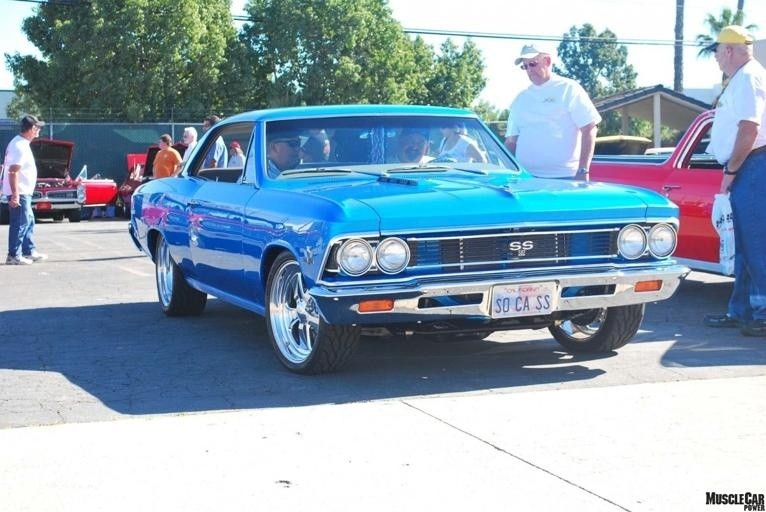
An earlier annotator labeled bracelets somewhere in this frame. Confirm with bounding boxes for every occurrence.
[722,162,739,175]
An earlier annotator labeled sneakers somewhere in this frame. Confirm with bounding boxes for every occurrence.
[741,319,766,337]
[21,251,47,262]
[703,311,752,328]
[6,255,33,265]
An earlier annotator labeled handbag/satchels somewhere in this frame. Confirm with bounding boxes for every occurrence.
[80,205,124,220]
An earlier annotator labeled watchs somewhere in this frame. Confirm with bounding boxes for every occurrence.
[577,167,589,175]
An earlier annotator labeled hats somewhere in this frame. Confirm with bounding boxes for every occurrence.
[515,43,551,65]
[229,142,240,149]
[705,25,753,50]
[20,115,45,130]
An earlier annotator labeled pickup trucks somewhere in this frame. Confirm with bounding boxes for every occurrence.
[575,107,735,279]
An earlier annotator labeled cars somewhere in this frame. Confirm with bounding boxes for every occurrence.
[129,104,690,376]
[116,142,189,219]
[0,137,117,222]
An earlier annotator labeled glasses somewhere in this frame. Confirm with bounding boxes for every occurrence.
[520,57,538,70]
[273,139,301,149]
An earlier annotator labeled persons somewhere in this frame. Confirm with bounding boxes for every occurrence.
[504,39,600,182]
[2,115,50,266]
[229,141,246,169]
[201,115,226,168]
[387,123,429,166]
[152,134,184,179]
[703,24,766,336]
[239,120,370,185]
[437,118,489,165]
[182,127,204,172]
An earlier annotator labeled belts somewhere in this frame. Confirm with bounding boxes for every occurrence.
[751,145,766,155]
